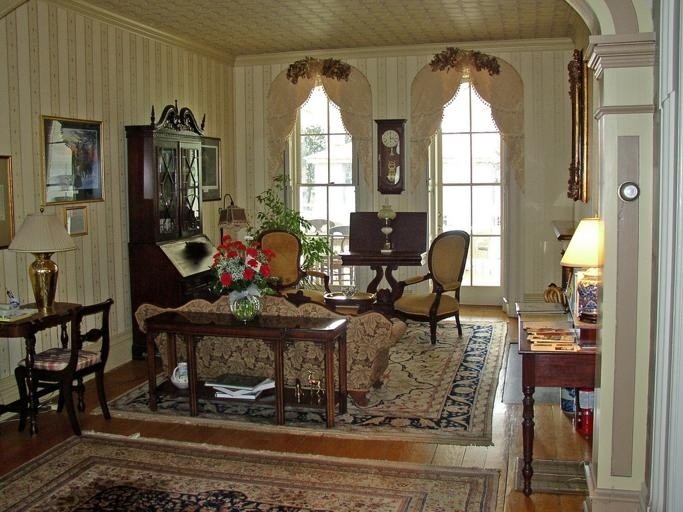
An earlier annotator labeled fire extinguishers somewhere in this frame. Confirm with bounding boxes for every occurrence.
[574,387,594,441]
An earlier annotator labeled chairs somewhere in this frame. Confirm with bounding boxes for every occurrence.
[393,231,470,344]
[15,299,114,436]
[257,229,331,304]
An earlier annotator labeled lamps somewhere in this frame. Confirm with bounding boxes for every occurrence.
[7,209,79,308]
[219,194,247,246]
[560,213,604,323]
[378,200,396,255]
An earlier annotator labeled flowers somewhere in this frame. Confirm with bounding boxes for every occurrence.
[208,235,278,322]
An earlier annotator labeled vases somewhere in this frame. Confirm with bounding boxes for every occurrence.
[229,285,262,323]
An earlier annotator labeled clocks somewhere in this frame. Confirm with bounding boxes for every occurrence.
[375,119,408,195]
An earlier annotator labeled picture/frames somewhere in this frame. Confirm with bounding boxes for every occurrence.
[0,154,15,249]
[201,137,222,201]
[566,50,588,203]
[41,114,106,206]
[64,205,89,237]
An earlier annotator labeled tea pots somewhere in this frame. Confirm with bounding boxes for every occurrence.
[171,362,188,389]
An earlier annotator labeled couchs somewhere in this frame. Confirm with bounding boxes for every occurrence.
[136,290,407,407]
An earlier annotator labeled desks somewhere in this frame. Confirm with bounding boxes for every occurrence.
[517,314,601,496]
[1,302,81,439]
[145,311,347,427]
[338,211,428,294]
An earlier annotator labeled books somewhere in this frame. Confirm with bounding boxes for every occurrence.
[205,372,277,401]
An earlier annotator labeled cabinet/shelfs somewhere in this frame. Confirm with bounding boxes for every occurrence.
[159,140,202,241]
[183,270,219,303]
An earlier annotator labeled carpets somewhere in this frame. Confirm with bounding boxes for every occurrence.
[88,321,507,445]
[0,431,501,512]
